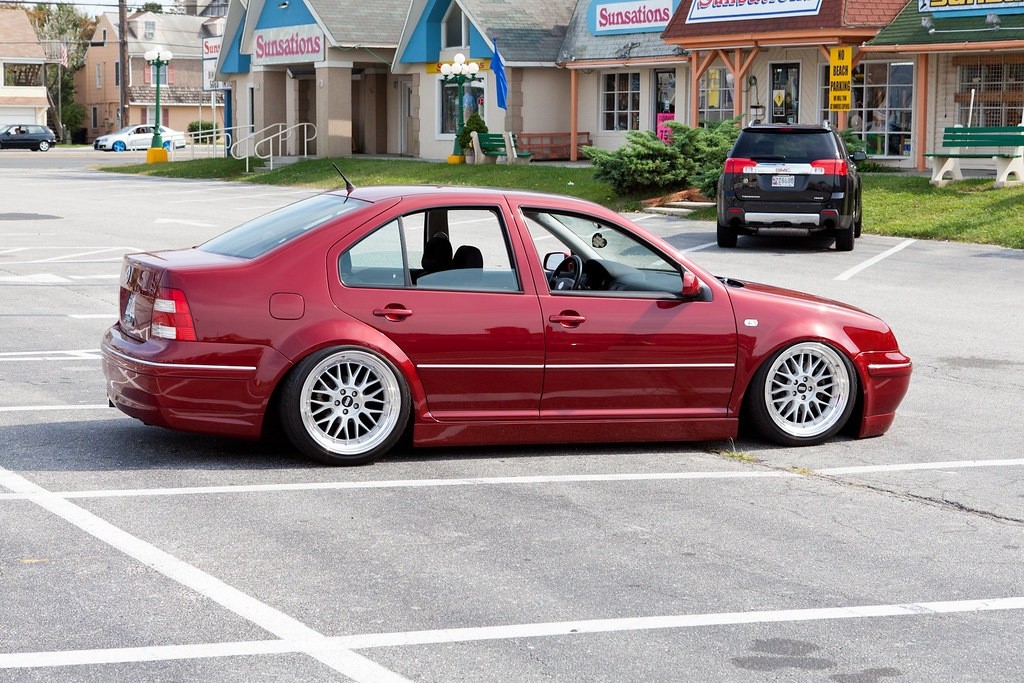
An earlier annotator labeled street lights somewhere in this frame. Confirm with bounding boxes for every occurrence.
[440,54,479,168]
[142,50,174,146]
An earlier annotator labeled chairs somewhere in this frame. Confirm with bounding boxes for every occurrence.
[417,232,453,280]
[20,131,25,134]
[453,245,483,285]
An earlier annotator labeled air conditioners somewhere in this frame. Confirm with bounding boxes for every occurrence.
[145,31,154,38]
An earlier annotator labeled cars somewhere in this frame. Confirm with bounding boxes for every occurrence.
[93,122,188,152]
[101,184,914,466]
[0,123,58,152]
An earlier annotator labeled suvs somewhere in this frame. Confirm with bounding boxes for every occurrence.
[716,118,866,252]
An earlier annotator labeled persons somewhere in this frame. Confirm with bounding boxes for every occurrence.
[140,129,145,134]
[15,128,21,134]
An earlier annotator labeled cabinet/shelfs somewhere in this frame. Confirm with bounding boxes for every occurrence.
[518,132,592,159]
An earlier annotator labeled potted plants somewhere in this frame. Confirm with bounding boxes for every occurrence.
[459,113,489,164]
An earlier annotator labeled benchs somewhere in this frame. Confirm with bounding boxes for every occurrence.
[470,131,535,165]
[285,226,365,284]
[922,124,1024,188]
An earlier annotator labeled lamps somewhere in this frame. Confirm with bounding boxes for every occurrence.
[562,51,576,62]
[920,17,936,35]
[277,1,288,8]
[985,14,1002,31]
